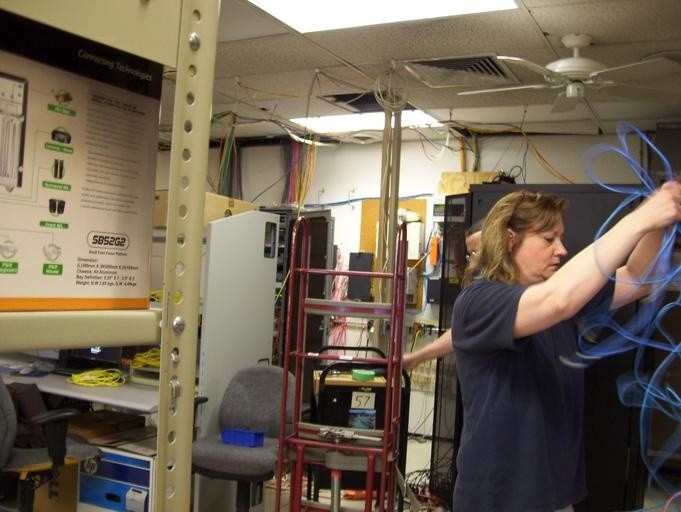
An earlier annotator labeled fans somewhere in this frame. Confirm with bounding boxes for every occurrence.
[458,32,681,114]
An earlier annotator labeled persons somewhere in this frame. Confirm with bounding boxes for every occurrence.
[451,180,680,512]
[401,216,486,371]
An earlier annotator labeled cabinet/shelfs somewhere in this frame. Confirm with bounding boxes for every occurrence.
[77,424,159,511]
[306,346,411,511]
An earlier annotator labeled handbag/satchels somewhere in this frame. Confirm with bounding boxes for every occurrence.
[7,382,49,449]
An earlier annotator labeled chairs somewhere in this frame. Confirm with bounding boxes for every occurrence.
[0,376,101,512]
[193,365,296,512]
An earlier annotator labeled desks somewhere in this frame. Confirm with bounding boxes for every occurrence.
[0,366,158,415]
